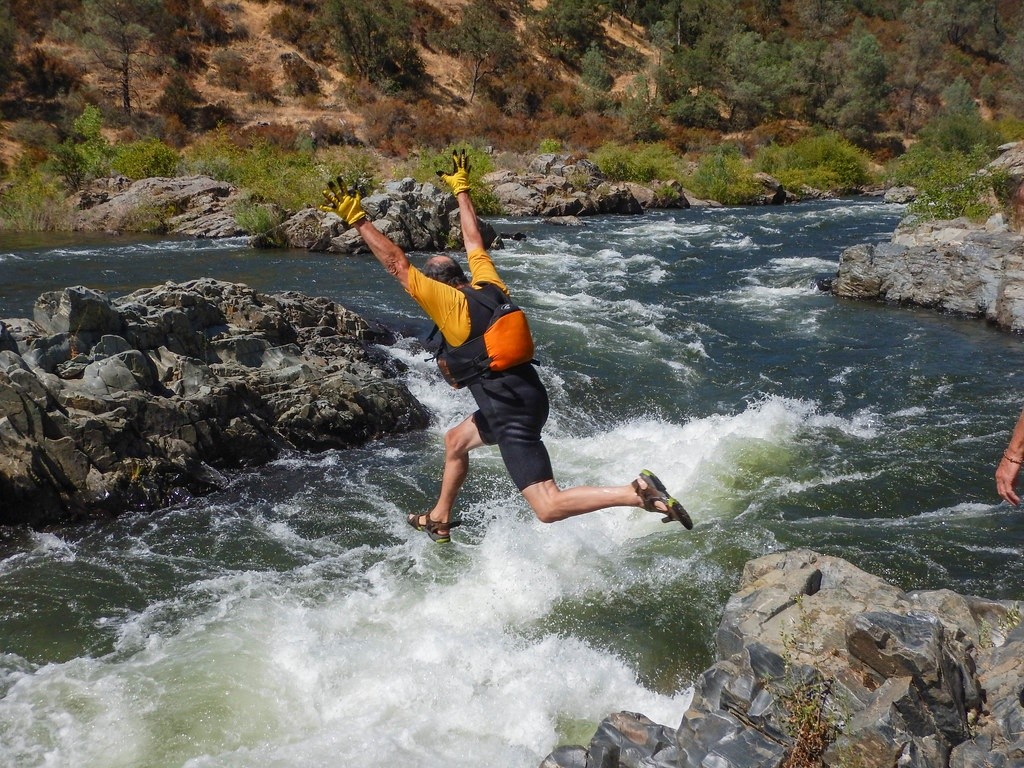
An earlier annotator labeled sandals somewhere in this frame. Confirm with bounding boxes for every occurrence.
[407,513,451,544]
[631,469,692,530]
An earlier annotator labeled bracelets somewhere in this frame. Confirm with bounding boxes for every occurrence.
[1004,448,1024,464]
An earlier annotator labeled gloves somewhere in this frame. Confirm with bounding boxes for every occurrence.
[436,148,472,196]
[319,177,367,226]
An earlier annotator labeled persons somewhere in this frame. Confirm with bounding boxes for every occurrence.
[320,151,693,545]
[994,407,1024,507]
